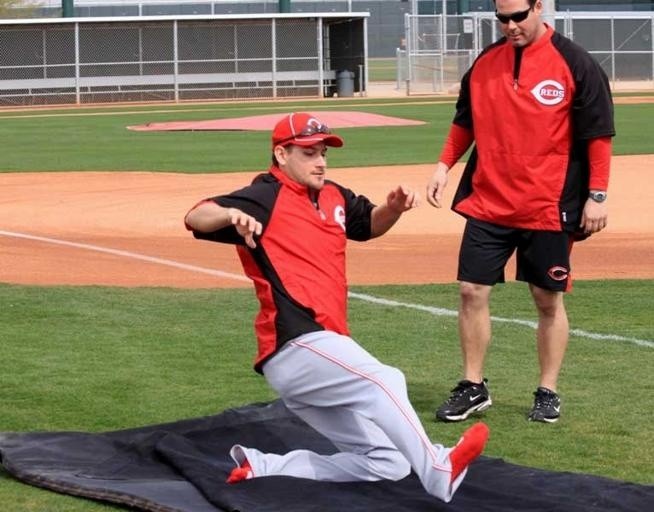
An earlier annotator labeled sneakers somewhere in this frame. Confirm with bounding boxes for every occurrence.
[436,379,492,420]
[529,386,560,422]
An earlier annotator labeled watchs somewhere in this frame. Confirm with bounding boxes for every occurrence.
[589,192,607,202]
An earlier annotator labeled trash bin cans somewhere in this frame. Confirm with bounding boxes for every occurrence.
[336,69,355,97]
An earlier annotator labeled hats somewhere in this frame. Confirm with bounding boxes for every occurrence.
[272,112,343,151]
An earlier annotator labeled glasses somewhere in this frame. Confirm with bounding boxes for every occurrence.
[496,7,532,24]
[274,124,330,144]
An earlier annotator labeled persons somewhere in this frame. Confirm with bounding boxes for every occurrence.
[184,112,491,502]
[426,0,616,422]
